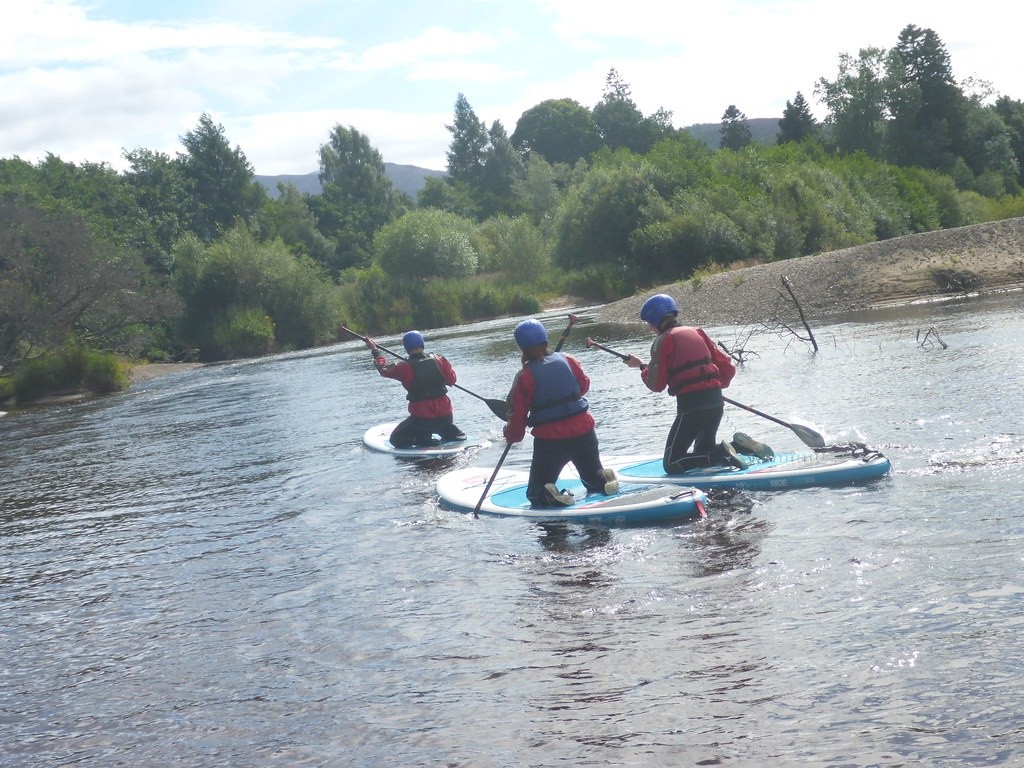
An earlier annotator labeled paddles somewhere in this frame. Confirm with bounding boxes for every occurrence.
[472,313,580,518]
[584,336,826,451]
[338,323,509,422]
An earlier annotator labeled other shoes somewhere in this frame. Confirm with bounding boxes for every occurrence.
[416,431,440,447]
[442,425,466,440]
[597,469,619,495]
[544,483,576,506]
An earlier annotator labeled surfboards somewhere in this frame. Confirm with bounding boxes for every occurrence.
[435,466,708,524]
[362,421,467,457]
[556,448,893,491]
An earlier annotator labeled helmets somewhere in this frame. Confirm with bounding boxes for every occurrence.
[514,319,548,350]
[402,330,425,354]
[640,294,679,327]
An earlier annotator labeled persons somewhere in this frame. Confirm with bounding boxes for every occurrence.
[503,318,618,506]
[362,330,467,449]
[622,293,773,470]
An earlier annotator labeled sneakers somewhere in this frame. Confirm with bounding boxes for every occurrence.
[731,432,775,461]
[715,439,749,471]
